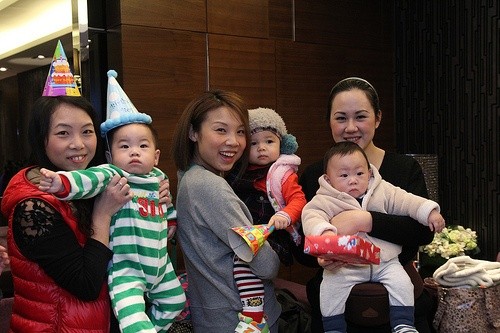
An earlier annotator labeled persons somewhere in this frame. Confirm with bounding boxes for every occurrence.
[298,74,445,333]
[169,88,307,333]
[2,39,187,333]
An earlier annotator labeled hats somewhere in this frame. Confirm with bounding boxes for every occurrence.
[101,68,153,136]
[246,106,298,154]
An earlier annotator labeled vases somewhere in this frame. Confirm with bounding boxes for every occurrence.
[426,254,456,278]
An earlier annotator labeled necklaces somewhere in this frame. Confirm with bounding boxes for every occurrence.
[167,191,170,196]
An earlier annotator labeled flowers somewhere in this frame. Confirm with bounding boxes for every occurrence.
[424,225,480,261]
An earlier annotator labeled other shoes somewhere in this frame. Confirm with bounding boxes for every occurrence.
[234,313,270,333]
[394,323,418,333]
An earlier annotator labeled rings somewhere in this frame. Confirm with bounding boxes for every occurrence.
[119,181,123,186]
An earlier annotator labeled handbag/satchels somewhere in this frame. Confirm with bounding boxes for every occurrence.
[422,277,500,332]
